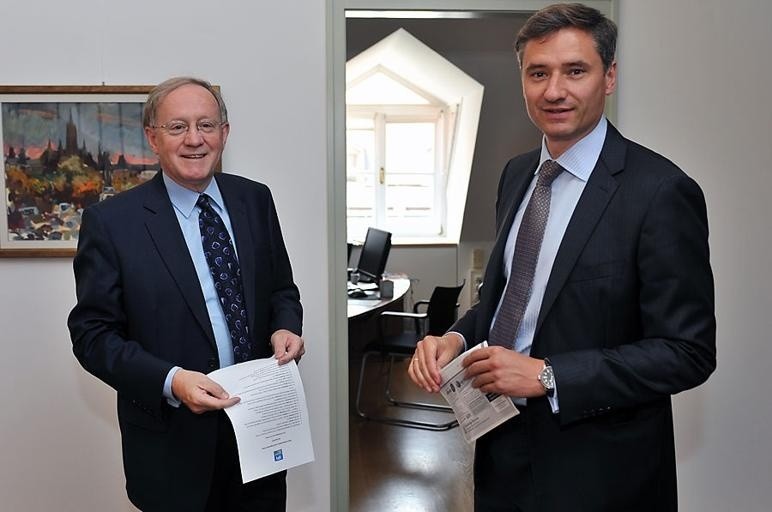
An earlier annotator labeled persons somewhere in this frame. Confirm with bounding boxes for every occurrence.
[67,78,306,512]
[407,3,716,512]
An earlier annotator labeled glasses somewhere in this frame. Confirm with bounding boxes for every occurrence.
[153,118,226,135]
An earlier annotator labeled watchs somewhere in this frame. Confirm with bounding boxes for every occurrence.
[537,358,554,397]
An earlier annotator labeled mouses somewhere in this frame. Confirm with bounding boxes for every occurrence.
[349,288,366,298]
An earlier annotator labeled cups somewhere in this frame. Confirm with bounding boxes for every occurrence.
[381,279,394,298]
[351,273,359,285]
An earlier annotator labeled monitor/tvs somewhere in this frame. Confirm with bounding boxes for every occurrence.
[357,227,392,292]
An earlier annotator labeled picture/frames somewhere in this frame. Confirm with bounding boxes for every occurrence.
[0,85,221,258]
[468,268,484,308]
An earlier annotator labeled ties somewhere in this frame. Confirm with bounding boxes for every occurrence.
[196,194,253,364]
[488,160,565,351]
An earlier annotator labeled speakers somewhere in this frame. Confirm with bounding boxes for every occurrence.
[379,280,393,298]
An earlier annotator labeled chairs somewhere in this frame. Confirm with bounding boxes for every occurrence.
[355,278,470,431]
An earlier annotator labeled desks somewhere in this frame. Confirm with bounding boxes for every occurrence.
[347,273,410,360]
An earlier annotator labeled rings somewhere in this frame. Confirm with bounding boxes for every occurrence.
[413,358,419,361]
